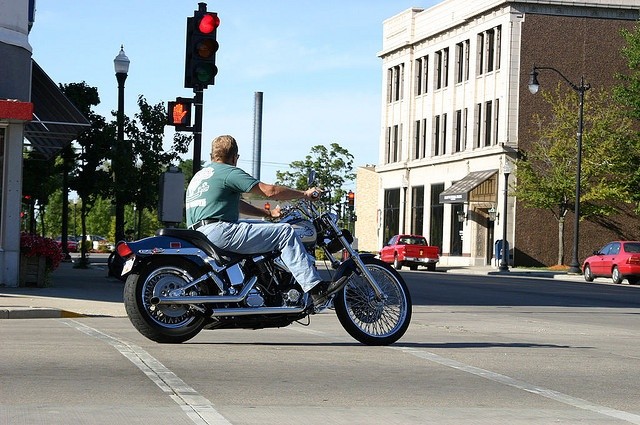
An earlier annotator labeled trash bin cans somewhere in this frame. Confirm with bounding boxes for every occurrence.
[495,239,509,267]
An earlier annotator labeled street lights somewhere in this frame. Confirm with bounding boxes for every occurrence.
[499,160,510,272]
[528,61,591,274]
[108,43,130,275]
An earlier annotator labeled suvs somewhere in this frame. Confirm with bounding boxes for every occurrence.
[77,235,116,251]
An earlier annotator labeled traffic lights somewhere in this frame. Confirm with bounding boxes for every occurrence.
[185,9,220,87]
[264,203,270,211]
[168,100,191,125]
[348,191,354,209]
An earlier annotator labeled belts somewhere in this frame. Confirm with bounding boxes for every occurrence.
[189,219,230,230]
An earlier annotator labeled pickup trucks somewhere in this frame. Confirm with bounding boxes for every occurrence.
[379,234,440,270]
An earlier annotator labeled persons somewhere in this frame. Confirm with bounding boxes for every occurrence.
[186,135,350,308]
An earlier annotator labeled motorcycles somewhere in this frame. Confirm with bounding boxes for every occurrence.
[116,170,412,344]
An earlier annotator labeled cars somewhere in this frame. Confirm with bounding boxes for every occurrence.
[56,236,78,252]
[582,240,640,284]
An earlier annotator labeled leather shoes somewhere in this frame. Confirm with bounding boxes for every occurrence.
[309,276,348,306]
